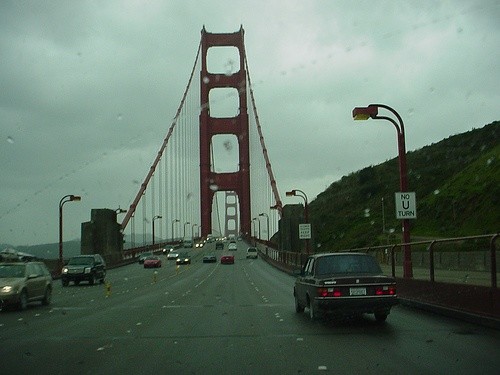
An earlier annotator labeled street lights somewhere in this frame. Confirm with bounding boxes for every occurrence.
[59,194,81,259]
[172,220,180,242]
[251,221,255,237]
[192,224,197,238]
[253,218,260,240]
[152,216,162,245]
[259,213,270,242]
[198,225,201,238]
[285,189,309,254]
[184,222,190,236]
[352,103,414,278]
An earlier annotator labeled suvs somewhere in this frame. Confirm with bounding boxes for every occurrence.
[61,254,106,287]
[247,247,258,258]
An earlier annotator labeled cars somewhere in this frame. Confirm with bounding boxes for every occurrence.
[144,256,162,267]
[139,252,153,263]
[0,260,54,311]
[184,234,243,264]
[163,244,191,264]
[294,252,398,325]
